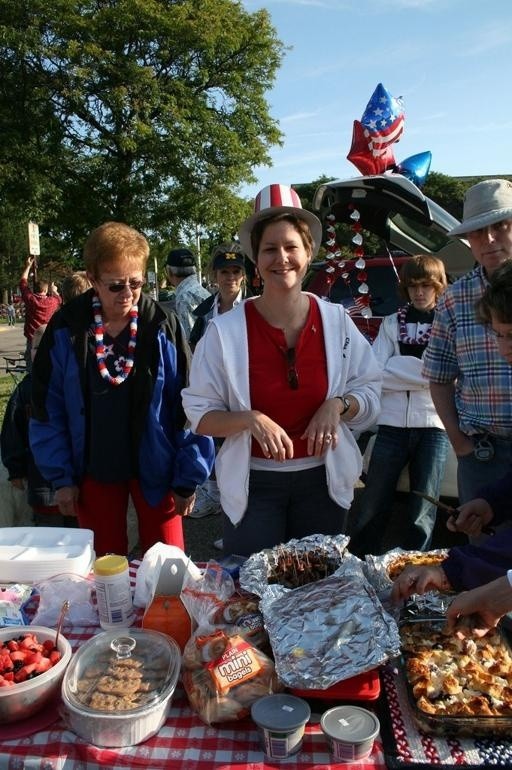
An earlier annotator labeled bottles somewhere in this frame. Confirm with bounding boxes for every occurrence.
[94,555,137,632]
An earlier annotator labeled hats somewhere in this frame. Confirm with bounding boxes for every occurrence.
[214,252,245,270]
[239,184,323,264]
[162,249,196,268]
[446,178,512,236]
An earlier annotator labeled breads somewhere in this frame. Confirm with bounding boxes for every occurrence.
[401,619,512,738]
[184,634,284,724]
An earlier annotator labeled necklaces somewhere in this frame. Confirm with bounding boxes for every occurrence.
[399,305,432,346]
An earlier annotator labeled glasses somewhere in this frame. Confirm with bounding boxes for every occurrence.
[287,347,298,388]
[98,276,147,292]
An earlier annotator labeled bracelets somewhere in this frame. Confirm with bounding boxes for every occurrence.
[334,396,351,414]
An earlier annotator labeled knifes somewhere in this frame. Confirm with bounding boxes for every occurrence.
[411,487,499,539]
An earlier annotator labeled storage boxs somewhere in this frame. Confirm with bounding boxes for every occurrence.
[285,670,381,714]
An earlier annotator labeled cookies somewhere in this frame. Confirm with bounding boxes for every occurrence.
[76,645,170,712]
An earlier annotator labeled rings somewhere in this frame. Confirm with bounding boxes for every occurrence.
[324,434,332,440]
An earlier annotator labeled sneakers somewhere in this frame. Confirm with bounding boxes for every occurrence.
[189,493,221,518]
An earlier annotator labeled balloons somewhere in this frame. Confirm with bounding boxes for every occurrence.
[361,83,406,158]
[391,150,432,191]
[346,119,397,177]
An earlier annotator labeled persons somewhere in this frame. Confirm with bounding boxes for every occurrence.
[29,221,216,557]
[6,259,93,370]
[347,252,448,557]
[422,178,511,550]
[392,258,512,602]
[440,569,512,643]
[2,325,56,509]
[156,249,246,519]
[180,186,384,564]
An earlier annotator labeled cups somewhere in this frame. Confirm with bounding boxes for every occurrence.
[320,705,378,761]
[250,693,311,759]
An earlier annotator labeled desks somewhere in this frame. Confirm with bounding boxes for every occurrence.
[0,559,512,769]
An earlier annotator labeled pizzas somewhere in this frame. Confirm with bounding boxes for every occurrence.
[388,554,447,577]
